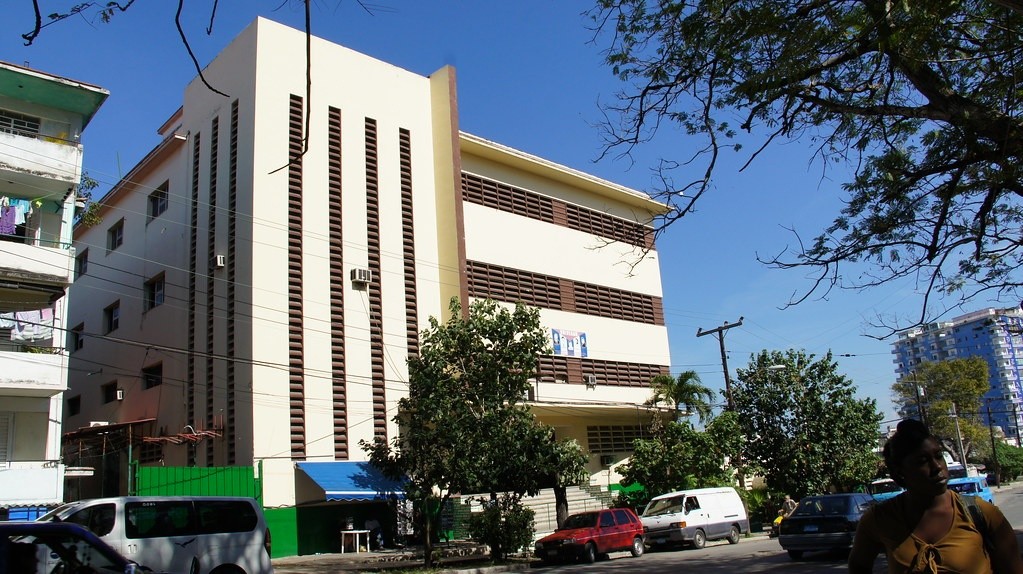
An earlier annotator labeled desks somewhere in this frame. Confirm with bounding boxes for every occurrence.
[341,529,371,554]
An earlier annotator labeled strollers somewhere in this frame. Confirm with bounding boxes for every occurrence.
[768,510,790,537]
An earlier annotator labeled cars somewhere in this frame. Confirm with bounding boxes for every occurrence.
[870,478,907,503]
[780,494,880,559]
[534,507,646,564]
[0,521,153,574]
[947,477,995,507]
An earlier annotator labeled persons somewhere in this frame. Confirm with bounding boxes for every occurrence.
[847,418,1023,574]
[783,495,796,515]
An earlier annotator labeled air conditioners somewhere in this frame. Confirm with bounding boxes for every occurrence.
[210,255,224,269]
[604,457,616,466]
[90,422,109,434]
[351,267,371,283]
[589,376,596,385]
[116,390,122,400]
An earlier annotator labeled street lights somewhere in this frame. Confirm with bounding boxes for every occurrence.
[949,414,969,477]
[916,387,927,421]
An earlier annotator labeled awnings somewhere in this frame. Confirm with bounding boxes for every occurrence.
[296,461,425,502]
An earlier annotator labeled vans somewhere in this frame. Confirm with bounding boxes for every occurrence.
[639,486,749,549]
[948,465,987,490]
[10,494,273,574]
